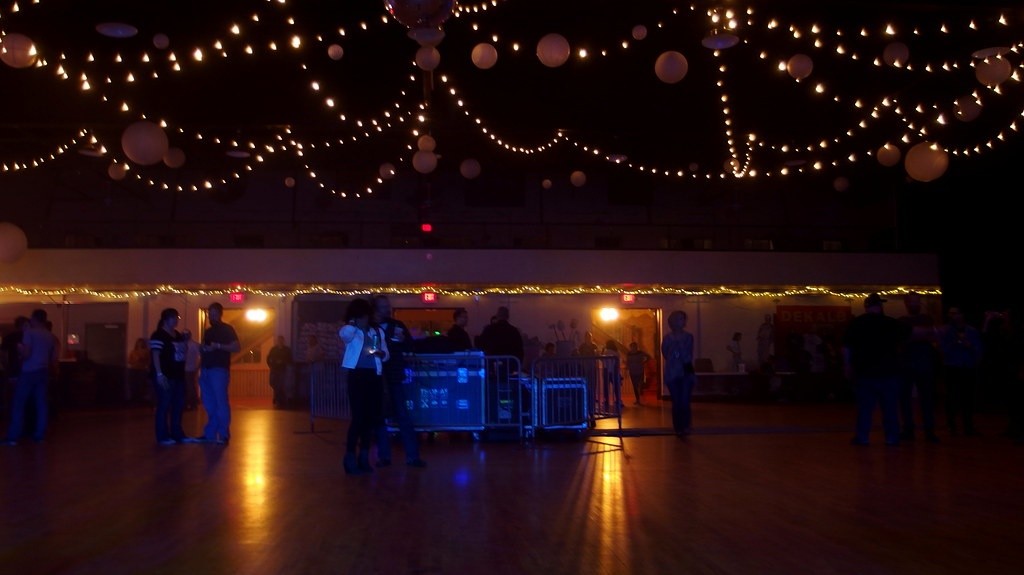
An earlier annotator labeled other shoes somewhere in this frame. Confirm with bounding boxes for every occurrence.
[850,439,867,445]
[216,439,225,445]
[406,459,427,468]
[193,437,217,444]
[949,427,958,438]
[157,438,177,447]
[0,438,17,447]
[900,424,916,441]
[375,459,391,467]
[923,425,939,444]
[963,421,984,438]
[177,436,192,443]
[343,449,375,474]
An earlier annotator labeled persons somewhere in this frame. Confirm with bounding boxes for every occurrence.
[445,306,524,382]
[266,335,293,408]
[303,335,326,364]
[661,311,696,440]
[541,332,652,409]
[726,331,743,370]
[842,293,986,445]
[128,302,241,446]
[338,295,428,475]
[0,309,60,447]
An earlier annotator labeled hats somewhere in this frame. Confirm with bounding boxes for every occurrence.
[864,293,882,307]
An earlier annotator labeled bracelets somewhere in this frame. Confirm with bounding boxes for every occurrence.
[157,373,162,376]
[217,344,220,349]
